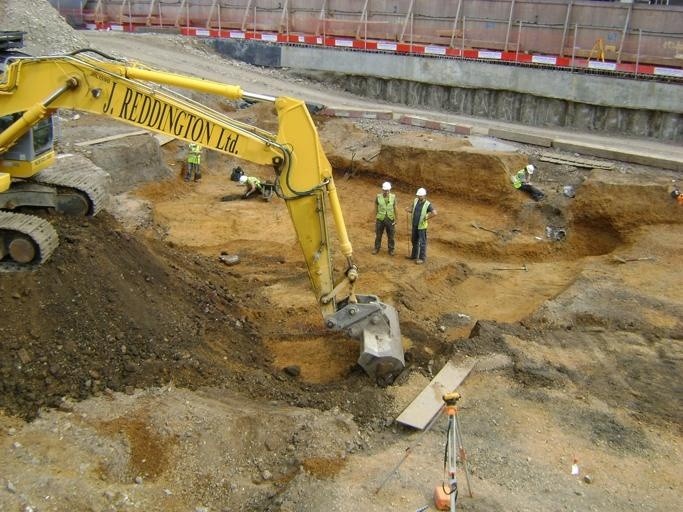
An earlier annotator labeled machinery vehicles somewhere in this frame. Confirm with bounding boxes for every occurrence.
[0,28,406,388]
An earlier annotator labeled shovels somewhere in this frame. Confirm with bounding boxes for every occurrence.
[362,151,380,163]
[194,155,201,181]
[614,255,652,263]
[471,221,498,233]
[343,152,356,181]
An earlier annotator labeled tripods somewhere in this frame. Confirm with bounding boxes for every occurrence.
[375,405,472,512]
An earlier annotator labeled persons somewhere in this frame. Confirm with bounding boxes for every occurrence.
[183,143,203,182]
[404,187,436,265]
[514,164,548,201]
[371,182,398,255]
[240,175,262,197]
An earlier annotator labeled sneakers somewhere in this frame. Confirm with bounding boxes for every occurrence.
[416,258,424,264]
[371,248,379,254]
[406,256,417,259]
[388,249,394,256]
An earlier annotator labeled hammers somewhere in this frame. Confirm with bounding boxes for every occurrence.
[493,264,528,272]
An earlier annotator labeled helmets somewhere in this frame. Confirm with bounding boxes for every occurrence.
[383,182,391,191]
[416,188,426,196]
[526,164,534,175]
[240,175,247,184]
